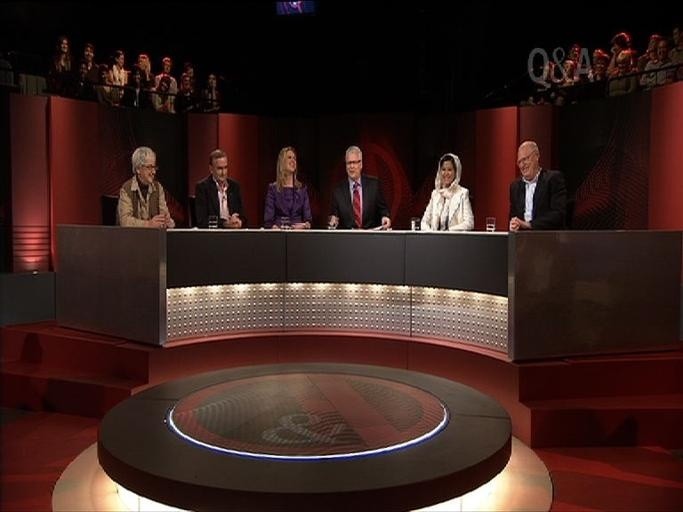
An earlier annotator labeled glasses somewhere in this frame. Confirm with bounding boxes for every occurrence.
[141,164,158,170]
[346,159,361,166]
[517,150,536,166]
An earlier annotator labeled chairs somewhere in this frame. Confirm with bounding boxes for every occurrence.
[100,194,119,225]
[189,195,196,227]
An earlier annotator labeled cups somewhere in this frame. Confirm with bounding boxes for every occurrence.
[485,216,495,230]
[208,216,218,228]
[326,214,337,229]
[280,216,292,229]
[411,216,420,230]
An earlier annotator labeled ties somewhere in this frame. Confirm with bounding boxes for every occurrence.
[220,187,228,219]
[353,181,362,229]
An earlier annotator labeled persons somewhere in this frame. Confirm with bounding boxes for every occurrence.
[524,26,681,106]
[114,147,177,230]
[194,150,247,228]
[51,36,219,111]
[325,144,392,230]
[262,147,314,230]
[507,141,568,231]
[416,153,476,234]
